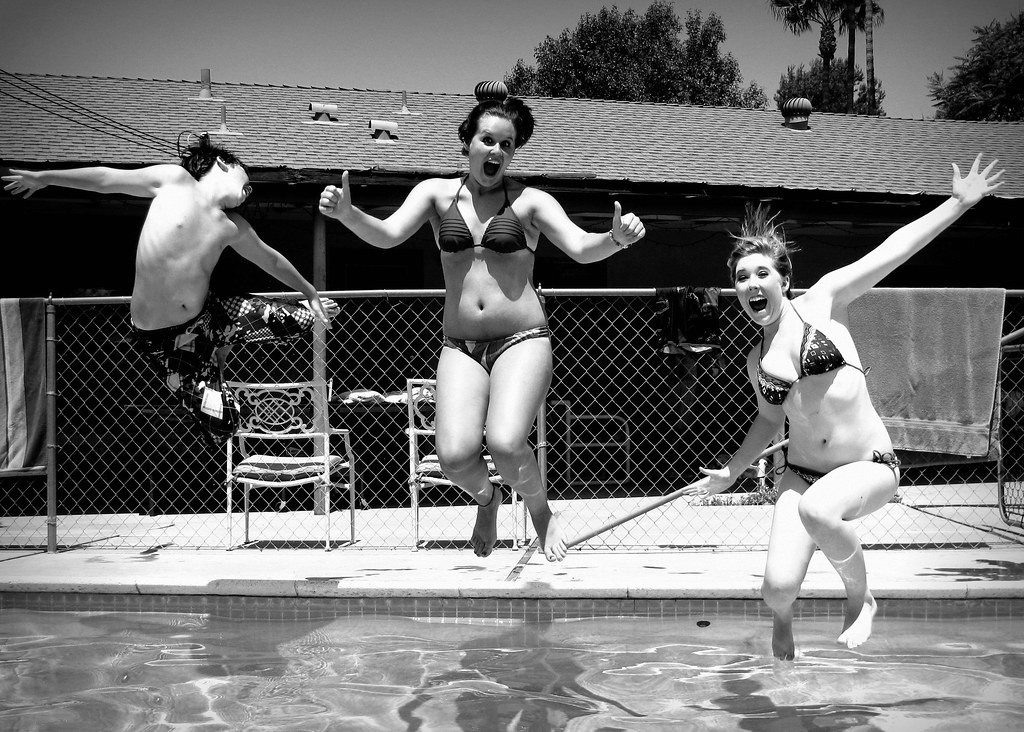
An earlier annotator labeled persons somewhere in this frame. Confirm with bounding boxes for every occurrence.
[682,152,1007,660]
[319,97,646,564]
[0,130,340,437]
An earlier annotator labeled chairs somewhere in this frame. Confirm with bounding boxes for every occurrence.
[223,381,354,545]
[407,379,547,556]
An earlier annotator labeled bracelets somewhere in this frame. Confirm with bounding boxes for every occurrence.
[609,230,630,250]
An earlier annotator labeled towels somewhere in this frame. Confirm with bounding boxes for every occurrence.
[833,288,1006,469]
[0,297,49,476]
